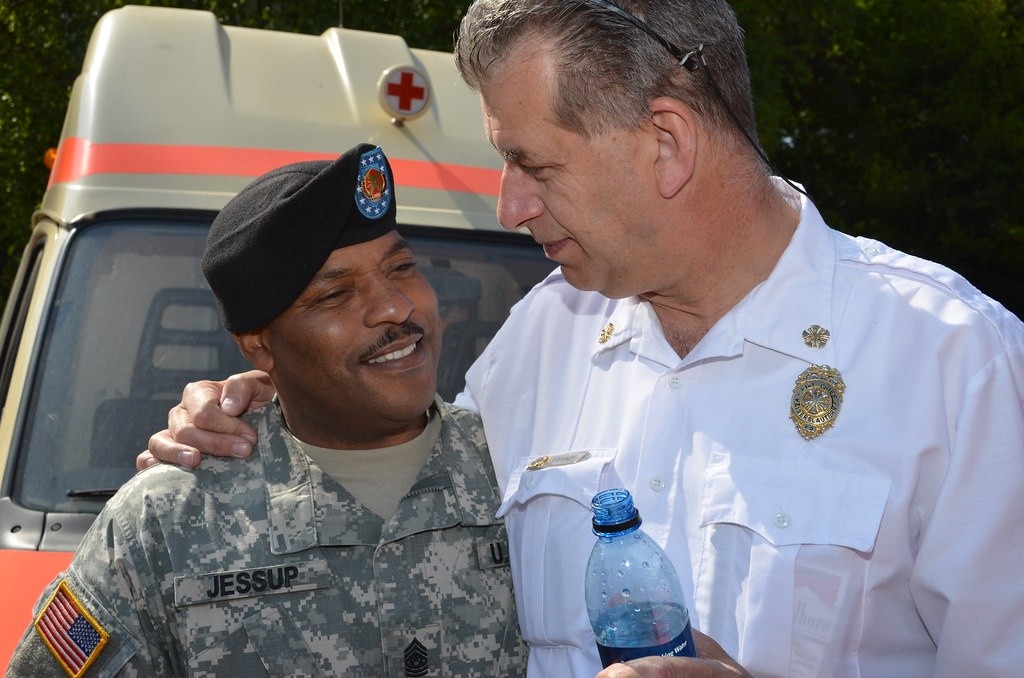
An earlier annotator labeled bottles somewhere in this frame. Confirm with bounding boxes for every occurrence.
[583,487,698,670]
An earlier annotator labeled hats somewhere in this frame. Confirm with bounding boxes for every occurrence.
[201,142,398,334]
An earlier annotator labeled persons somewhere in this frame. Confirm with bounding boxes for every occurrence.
[136,1,1024,678]
[3,143,530,678]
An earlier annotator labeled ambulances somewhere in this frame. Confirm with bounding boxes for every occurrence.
[0,6,566,678]
